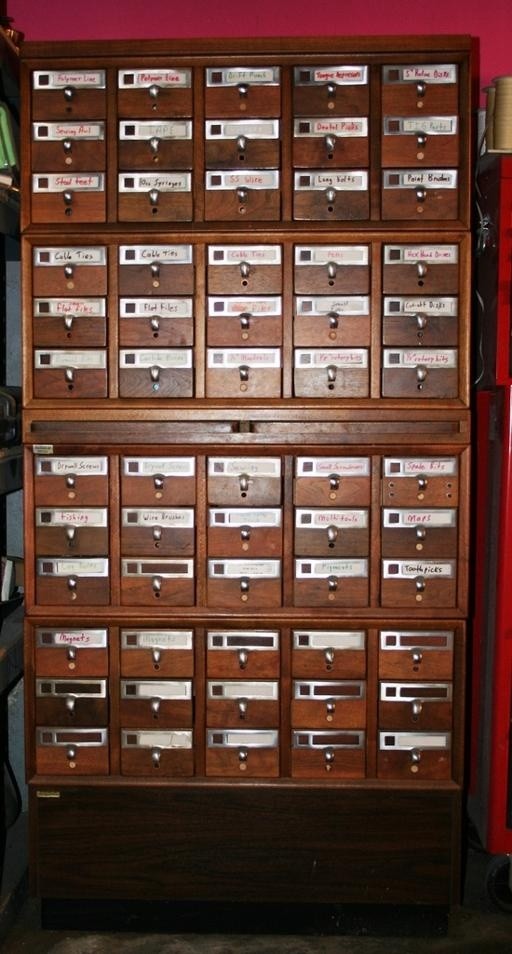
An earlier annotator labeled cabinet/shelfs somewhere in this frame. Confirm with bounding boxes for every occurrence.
[22,31,481,930]
